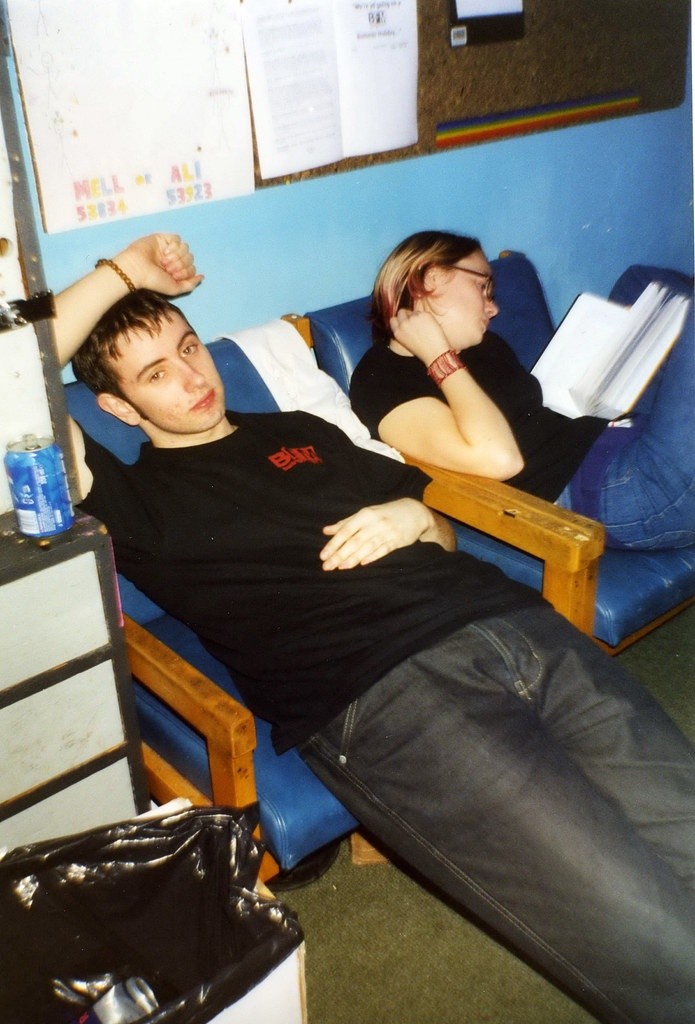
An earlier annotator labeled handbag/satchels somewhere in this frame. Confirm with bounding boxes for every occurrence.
[0,805,306,1024]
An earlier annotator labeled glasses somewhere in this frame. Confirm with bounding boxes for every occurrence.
[439,262,495,300]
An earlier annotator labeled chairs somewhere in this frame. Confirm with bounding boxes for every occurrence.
[63,313,592,886]
[279,250,695,656]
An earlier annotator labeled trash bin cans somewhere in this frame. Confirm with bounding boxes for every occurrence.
[0,795,309,1024]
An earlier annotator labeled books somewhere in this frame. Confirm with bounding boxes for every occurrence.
[528,279,691,422]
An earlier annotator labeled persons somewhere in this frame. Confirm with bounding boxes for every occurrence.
[49,230,693,1024]
[349,231,694,551]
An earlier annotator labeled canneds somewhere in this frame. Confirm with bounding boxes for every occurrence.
[3,434,75,538]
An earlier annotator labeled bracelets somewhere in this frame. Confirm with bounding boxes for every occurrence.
[95,259,136,297]
[426,350,465,387]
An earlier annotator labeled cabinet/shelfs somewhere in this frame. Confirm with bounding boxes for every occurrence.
[0,509,149,862]
